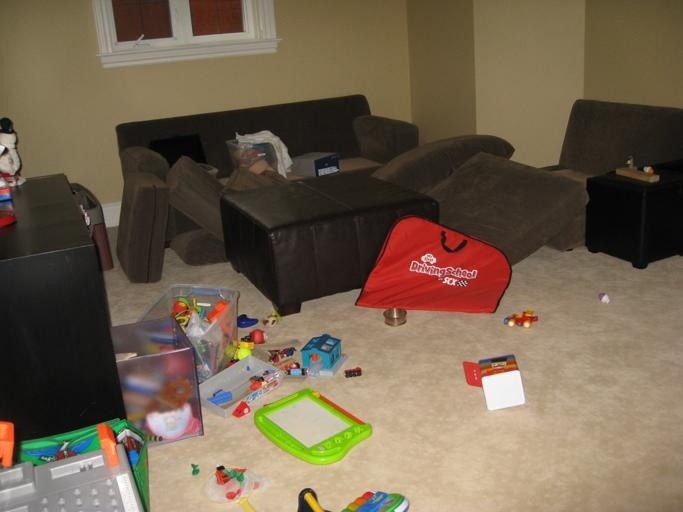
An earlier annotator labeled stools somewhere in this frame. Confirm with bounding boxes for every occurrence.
[221,172,440,315]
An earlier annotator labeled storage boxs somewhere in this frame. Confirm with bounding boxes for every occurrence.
[110,317,203,449]
[290,151,341,175]
[136,283,239,384]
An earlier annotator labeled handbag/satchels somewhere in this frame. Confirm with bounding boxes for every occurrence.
[355,214,511,313]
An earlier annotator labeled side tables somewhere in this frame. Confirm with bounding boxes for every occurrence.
[582,164,683,272]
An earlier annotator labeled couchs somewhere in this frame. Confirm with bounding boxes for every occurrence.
[540,98,682,272]
[116,95,418,247]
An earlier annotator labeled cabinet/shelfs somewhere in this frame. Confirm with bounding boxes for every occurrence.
[0,174,126,442]
